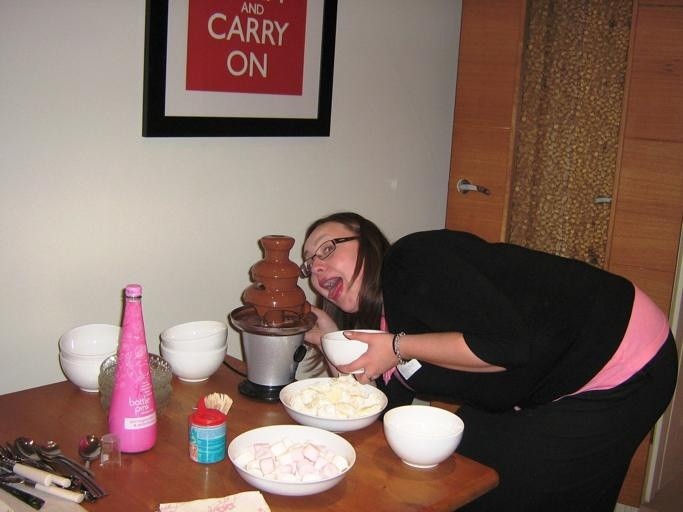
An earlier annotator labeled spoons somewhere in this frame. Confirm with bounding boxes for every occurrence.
[0,434,109,511]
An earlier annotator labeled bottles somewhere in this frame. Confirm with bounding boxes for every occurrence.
[107,285,159,454]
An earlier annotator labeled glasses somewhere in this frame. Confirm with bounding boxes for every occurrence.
[298,235,364,279]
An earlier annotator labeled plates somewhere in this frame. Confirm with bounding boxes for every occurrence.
[280,377,388,433]
[227,425,355,496]
[98,353,173,419]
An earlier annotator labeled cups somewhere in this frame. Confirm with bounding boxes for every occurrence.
[100,435,121,469]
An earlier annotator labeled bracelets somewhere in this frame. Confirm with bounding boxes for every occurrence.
[392,331,408,365]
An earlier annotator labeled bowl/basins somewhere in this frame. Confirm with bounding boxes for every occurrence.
[159,343,227,382]
[159,321,227,350]
[383,406,464,468]
[322,329,384,374]
[57,356,102,392]
[58,324,121,360]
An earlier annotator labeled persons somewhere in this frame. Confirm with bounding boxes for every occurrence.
[300,212,678,511]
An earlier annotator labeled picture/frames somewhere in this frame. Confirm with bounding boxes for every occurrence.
[142,2,338,139]
